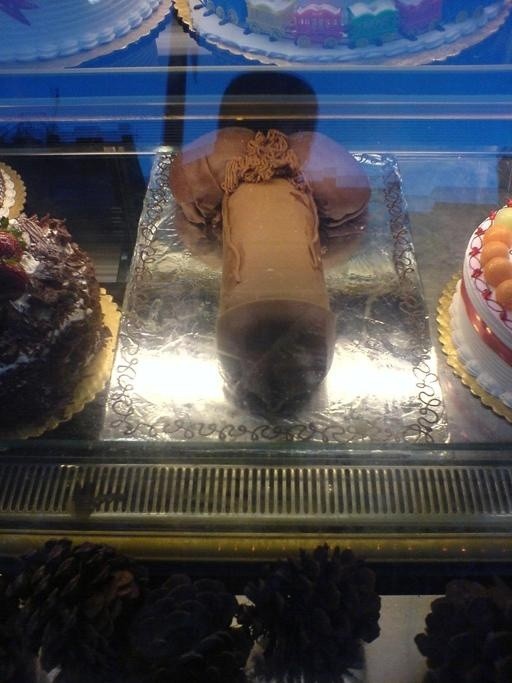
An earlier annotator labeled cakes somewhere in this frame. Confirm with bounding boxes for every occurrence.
[447,198,512,407]
[0,167,17,219]
[188,0,510,64]
[170,127,371,414]
[0,212,103,429]
[1,0,167,65]
